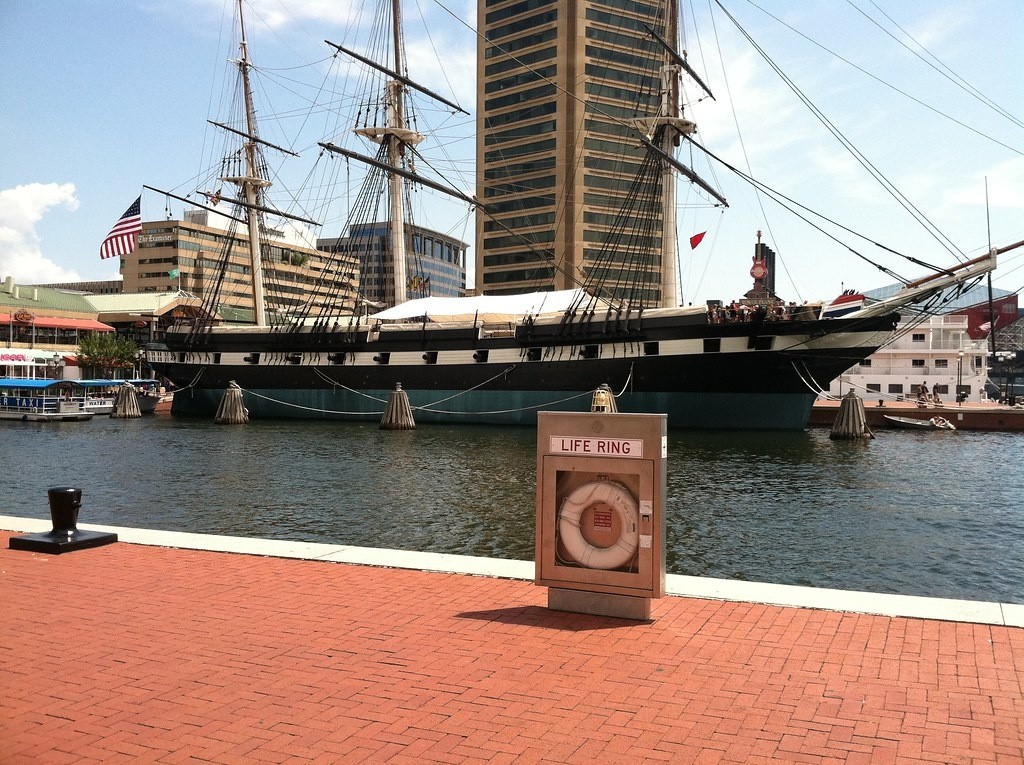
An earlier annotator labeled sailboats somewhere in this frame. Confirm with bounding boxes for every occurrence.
[145,1,1024,431]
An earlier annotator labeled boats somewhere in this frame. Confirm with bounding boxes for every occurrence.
[0,379,95,421]
[883,414,956,430]
[74,378,162,414]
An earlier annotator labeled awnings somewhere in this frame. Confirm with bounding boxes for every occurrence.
[0,313,115,331]
[64,355,133,366]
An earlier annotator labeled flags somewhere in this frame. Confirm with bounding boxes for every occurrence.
[690,231,708,249]
[210,189,221,205]
[421,276,429,290]
[100,195,143,259]
[168,268,179,280]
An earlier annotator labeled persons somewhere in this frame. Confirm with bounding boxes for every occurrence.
[689,302,692,306]
[707,300,808,323]
[65,390,70,401]
[917,381,929,400]
